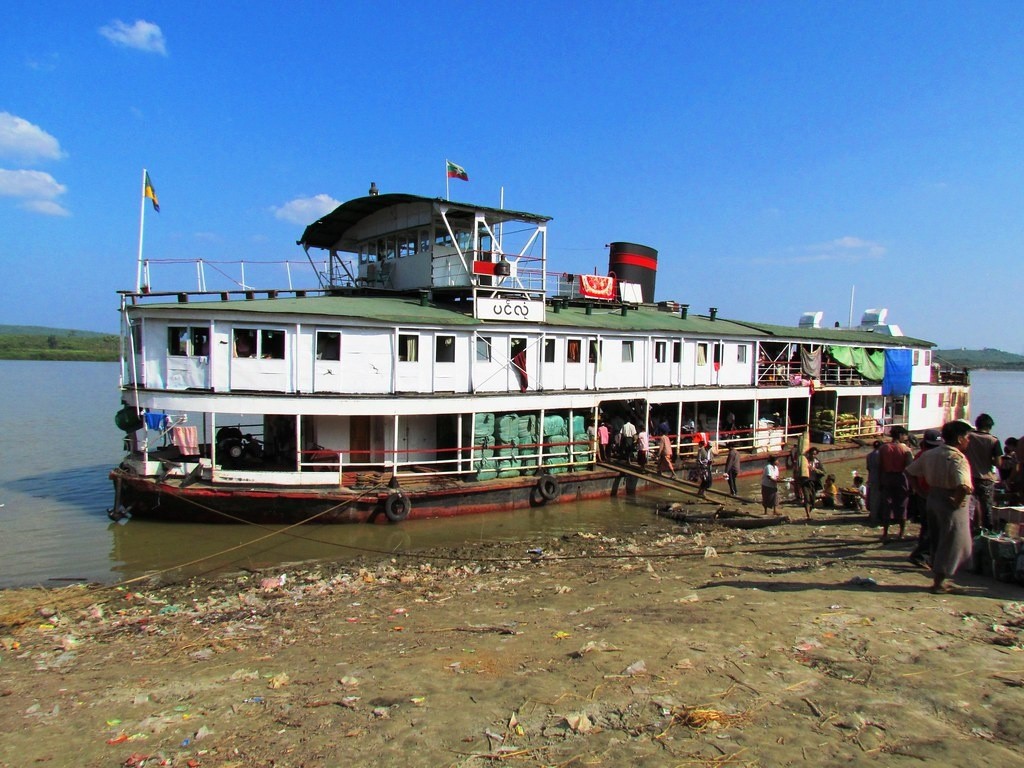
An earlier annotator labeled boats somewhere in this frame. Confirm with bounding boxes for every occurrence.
[109,192,971,524]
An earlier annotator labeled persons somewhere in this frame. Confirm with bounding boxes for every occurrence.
[636,424,649,470]
[757,351,801,386]
[696,440,714,496]
[726,410,735,427]
[796,447,827,519]
[814,473,837,507]
[643,415,695,435]
[759,454,782,516]
[864,412,1024,595]
[838,476,867,511]
[584,415,636,466]
[655,432,677,479]
[760,409,791,427]
[724,441,741,495]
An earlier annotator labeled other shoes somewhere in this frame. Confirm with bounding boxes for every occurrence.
[906,557,930,570]
[898,535,906,542]
[974,526,982,535]
[879,536,890,542]
[856,508,862,513]
[774,511,782,515]
[935,584,967,594]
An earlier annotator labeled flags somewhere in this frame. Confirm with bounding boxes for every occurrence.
[447,161,469,182]
[145,171,160,213]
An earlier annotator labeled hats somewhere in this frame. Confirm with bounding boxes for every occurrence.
[924,430,942,446]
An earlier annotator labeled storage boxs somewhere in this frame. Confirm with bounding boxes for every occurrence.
[136,462,222,480]
[812,431,830,444]
[981,506,1024,586]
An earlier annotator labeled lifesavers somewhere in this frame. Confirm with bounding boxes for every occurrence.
[537,476,560,500]
[385,493,412,521]
